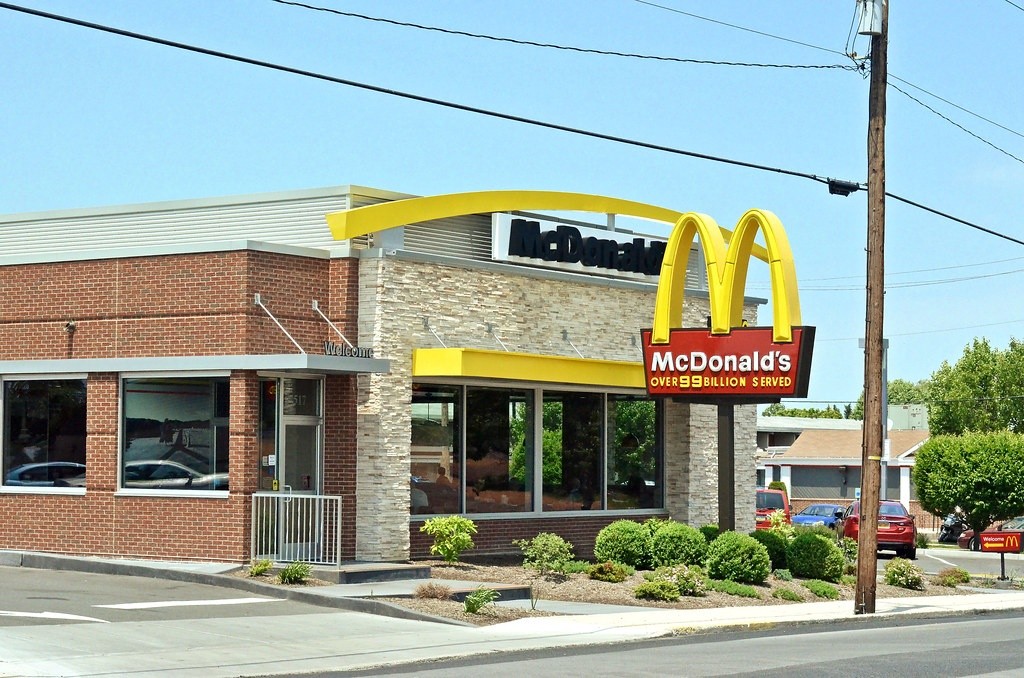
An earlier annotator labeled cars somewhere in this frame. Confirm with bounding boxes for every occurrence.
[3,460,87,488]
[957,516,1024,552]
[791,504,849,540]
[124,459,228,489]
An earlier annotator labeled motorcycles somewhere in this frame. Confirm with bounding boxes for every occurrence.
[937,512,969,543]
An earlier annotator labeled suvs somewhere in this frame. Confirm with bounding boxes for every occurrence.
[842,498,918,560]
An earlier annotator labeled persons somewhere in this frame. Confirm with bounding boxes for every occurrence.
[436,467,455,488]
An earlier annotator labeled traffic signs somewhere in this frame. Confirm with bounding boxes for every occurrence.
[979,531,1024,555]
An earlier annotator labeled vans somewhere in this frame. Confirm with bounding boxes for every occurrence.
[755,487,793,534]
